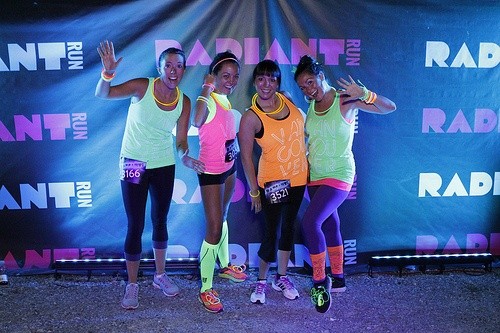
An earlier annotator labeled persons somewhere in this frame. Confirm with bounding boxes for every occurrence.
[238,59,309,304]
[294,54,396,314]
[94,40,206,309]
[192,50,249,313]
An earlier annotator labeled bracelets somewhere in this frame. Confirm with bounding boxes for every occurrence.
[360,86,377,104]
[101,69,115,82]
[249,190,260,197]
[202,81,215,91]
[197,96,209,105]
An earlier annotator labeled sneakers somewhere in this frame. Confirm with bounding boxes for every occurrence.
[122,283,139,308]
[251,279,267,303]
[198,287,223,312]
[310,275,332,313]
[272,275,299,299]
[218,265,246,282]
[327,274,346,292]
[152,273,180,296]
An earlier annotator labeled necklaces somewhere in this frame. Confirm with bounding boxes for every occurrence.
[211,93,231,111]
[312,86,338,114]
[151,77,179,107]
[252,91,285,114]
